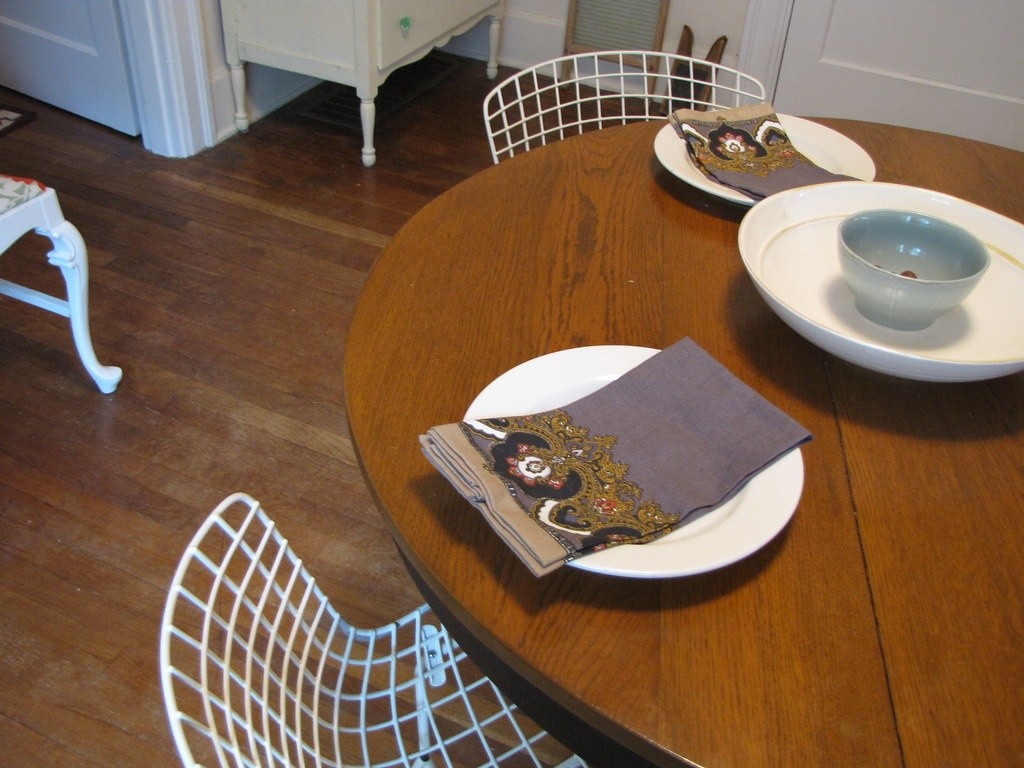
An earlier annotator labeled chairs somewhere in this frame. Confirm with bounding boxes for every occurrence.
[478,50,767,164]
[157,486,595,768]
[0,174,122,397]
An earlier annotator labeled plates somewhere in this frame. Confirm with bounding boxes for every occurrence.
[738,180,1024,387]
[459,345,805,577]
[654,112,875,209]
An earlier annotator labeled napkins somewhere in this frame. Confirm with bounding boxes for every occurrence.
[669,102,869,202]
[419,335,812,580]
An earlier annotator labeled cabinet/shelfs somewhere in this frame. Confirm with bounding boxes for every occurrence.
[219,0,507,168]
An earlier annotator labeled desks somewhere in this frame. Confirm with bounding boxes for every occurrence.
[340,107,1024,768]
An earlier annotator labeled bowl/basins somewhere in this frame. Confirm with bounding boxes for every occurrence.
[837,208,991,332]
